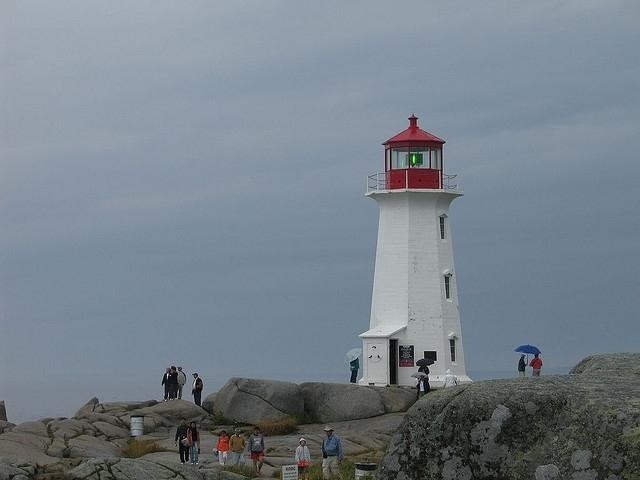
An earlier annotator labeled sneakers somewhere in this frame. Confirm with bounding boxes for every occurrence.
[180,458,199,466]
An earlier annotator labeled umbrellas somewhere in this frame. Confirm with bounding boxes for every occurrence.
[513,343,541,365]
[410,372,426,377]
[344,347,362,362]
[415,358,434,367]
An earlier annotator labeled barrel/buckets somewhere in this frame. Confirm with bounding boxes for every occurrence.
[130,415,145,437]
[354,462,379,480]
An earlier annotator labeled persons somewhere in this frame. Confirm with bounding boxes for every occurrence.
[518,353,528,376]
[216,430,229,467]
[294,437,311,480]
[416,377,431,401]
[350,358,359,384]
[321,426,344,480]
[186,421,200,465]
[177,367,186,400]
[161,368,175,402]
[169,366,178,400]
[529,354,542,376]
[229,428,246,472]
[174,416,189,464]
[418,366,429,382]
[192,373,203,407]
[247,426,265,475]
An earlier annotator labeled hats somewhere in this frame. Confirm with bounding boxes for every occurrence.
[520,354,526,358]
[299,438,307,445]
[323,424,335,431]
[177,366,182,369]
[192,373,198,376]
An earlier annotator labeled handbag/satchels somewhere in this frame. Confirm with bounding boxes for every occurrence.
[351,365,357,370]
[322,448,328,459]
[181,437,190,447]
